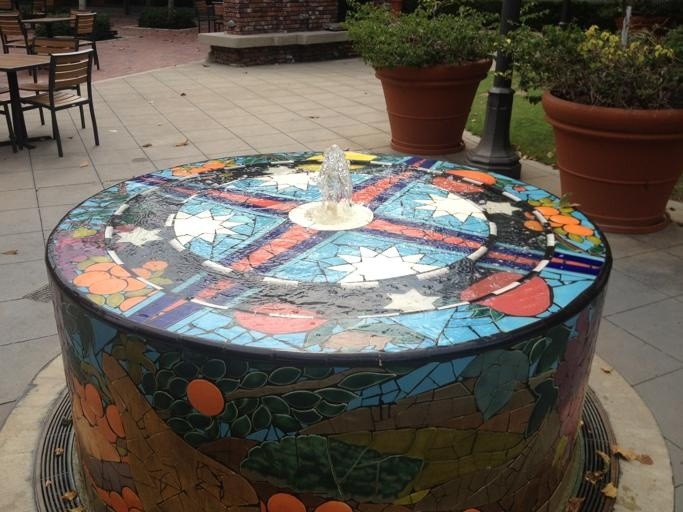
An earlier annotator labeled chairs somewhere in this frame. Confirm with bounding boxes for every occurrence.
[1,9,101,157]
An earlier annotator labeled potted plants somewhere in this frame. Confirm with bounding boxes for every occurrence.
[493,1,683,235]
[343,0,501,155]
